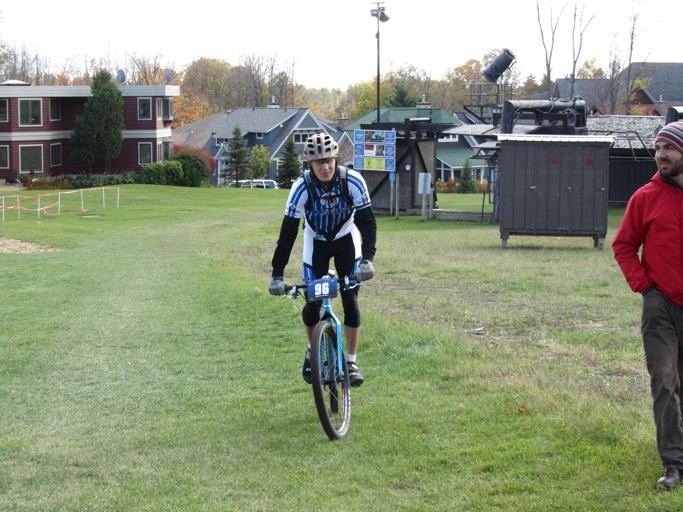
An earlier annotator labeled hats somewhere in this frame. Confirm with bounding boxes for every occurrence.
[655,118,683,154]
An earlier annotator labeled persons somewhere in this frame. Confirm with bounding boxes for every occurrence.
[611,118,683,492]
[268,132,376,386]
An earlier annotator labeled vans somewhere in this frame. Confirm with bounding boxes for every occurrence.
[229,179,279,189]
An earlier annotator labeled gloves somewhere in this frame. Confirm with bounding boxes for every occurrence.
[352,259,374,283]
[268,277,290,295]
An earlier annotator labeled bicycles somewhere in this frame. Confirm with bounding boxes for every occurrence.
[285,273,364,441]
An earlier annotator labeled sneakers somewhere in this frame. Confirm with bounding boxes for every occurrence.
[303,347,313,384]
[657,468,681,487]
[347,361,363,386]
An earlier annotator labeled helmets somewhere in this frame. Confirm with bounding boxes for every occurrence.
[303,133,339,162]
